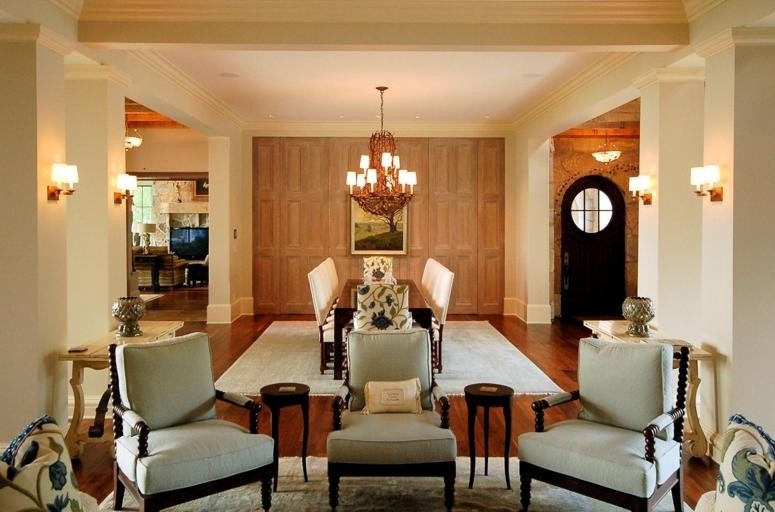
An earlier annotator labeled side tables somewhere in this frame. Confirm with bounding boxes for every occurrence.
[259,383,311,492]
[465,384,515,490]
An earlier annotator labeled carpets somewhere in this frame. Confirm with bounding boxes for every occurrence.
[215,320,565,398]
[94,455,693,512]
[140,294,164,305]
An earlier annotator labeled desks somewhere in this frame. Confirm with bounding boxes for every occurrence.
[584,319,710,458]
[335,278,433,381]
[136,254,161,294]
[57,321,184,445]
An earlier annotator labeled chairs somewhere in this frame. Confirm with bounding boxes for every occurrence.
[108,331,278,512]
[354,284,412,330]
[364,256,392,285]
[328,327,457,511]
[517,337,691,512]
[308,257,348,375]
[421,257,454,374]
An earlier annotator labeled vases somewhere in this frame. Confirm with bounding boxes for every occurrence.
[620,294,656,337]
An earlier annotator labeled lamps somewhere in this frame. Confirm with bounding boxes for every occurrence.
[124,114,143,151]
[137,223,156,254]
[47,164,79,200]
[691,164,722,202]
[345,87,417,217]
[630,174,652,205]
[592,121,622,162]
[114,174,138,204]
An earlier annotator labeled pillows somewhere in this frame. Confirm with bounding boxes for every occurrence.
[359,378,424,414]
[0,415,81,512]
[714,413,774,512]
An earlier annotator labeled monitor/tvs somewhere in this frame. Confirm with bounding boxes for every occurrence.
[170,226,209,260]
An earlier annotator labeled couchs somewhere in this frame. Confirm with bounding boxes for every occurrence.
[132,245,186,286]
[187,254,208,287]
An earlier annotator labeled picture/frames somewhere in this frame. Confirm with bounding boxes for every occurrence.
[348,184,409,258]
[191,179,209,202]
